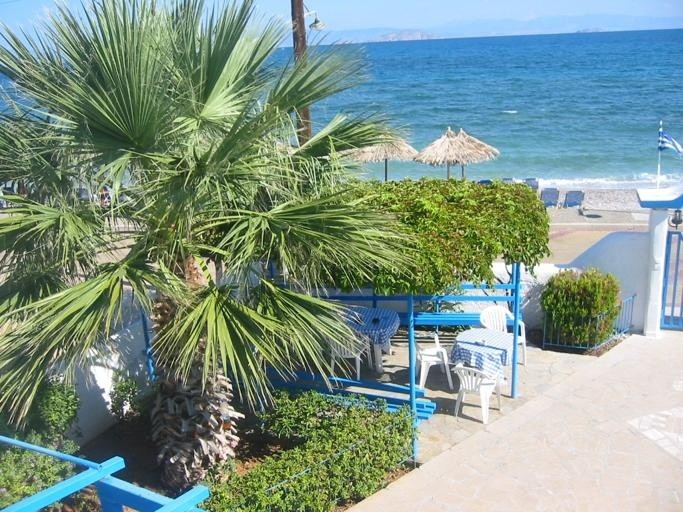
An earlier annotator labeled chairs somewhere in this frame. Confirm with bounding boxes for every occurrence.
[407,304,527,426]
[478,177,585,209]
[327,329,373,382]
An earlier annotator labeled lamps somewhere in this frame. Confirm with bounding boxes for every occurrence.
[667,207,683,231]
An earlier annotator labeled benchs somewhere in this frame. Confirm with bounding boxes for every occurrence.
[267,363,437,460]
[312,283,523,336]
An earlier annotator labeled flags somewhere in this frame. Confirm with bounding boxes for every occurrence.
[657,120,683,158]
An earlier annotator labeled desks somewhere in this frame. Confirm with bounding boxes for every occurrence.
[337,306,400,373]
